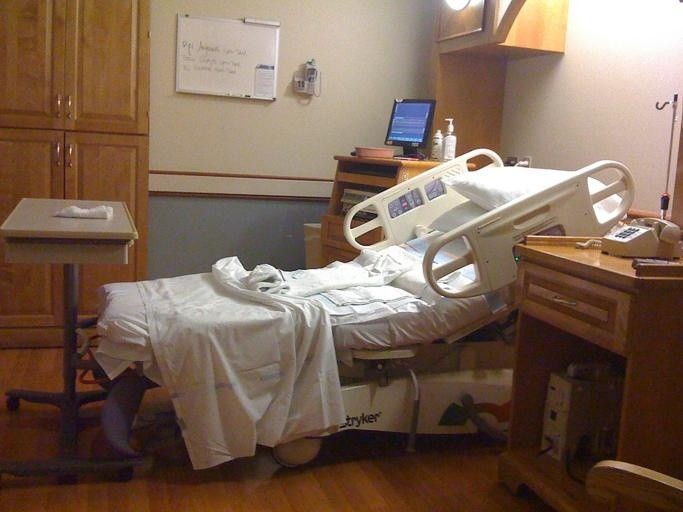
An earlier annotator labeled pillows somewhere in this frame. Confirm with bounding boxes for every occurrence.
[427,165,634,240]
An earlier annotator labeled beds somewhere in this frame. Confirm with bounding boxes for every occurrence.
[91,148,638,471]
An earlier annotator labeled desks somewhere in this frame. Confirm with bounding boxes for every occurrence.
[0,196,144,476]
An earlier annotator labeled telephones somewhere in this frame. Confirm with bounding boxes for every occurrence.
[600,216,682,258]
[305,67,319,97]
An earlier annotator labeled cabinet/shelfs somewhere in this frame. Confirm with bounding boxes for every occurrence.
[0,1,151,350]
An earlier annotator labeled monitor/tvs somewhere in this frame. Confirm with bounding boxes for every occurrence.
[385,98,436,159]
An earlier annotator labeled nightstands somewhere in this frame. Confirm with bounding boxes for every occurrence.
[320,154,446,268]
[498,234,683,512]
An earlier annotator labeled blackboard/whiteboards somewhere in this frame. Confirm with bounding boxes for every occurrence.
[175,11,281,101]
